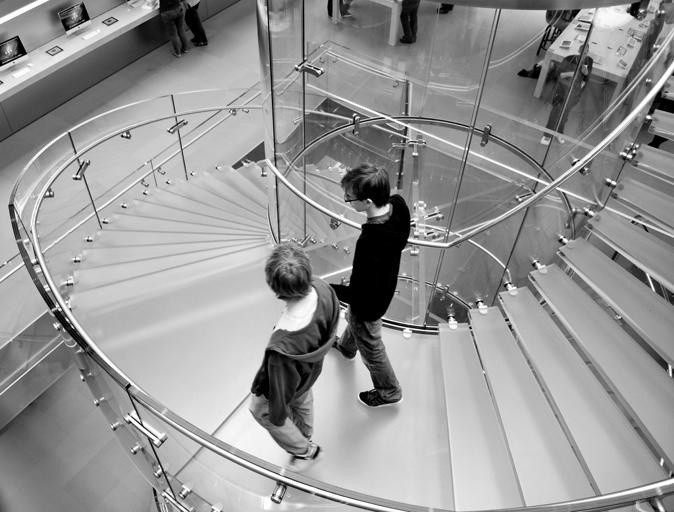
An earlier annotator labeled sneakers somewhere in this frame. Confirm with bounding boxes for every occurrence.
[331,336,356,361]
[283,441,322,473]
[357,387,402,408]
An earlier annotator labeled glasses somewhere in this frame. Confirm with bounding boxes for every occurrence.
[342,193,359,203]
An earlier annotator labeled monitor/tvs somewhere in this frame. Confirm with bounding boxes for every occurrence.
[0,36,29,72]
[57,1,92,36]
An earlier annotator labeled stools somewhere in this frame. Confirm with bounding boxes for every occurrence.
[536,24,562,56]
[589,75,610,130]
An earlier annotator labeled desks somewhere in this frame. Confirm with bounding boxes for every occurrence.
[533,1,658,123]
[0,0,241,141]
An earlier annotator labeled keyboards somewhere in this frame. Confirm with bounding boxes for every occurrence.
[131,0,145,8]
[12,67,31,79]
[83,30,98,41]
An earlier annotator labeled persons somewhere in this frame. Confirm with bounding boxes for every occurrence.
[250,243,341,474]
[647,92,674,150]
[145,0,208,59]
[399,0,420,44]
[540,43,594,146]
[328,162,412,409]
[327,0,352,21]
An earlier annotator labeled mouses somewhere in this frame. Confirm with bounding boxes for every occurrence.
[28,63,32,66]
[96,29,101,32]
